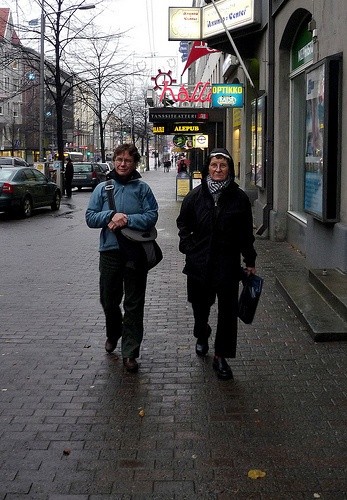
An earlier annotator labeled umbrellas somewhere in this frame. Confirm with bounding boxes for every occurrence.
[176,158,191,167]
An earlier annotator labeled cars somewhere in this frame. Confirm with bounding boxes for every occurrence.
[63,162,108,192]
[0,155,62,218]
[64,152,84,163]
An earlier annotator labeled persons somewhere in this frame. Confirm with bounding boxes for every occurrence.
[175,148,259,379]
[85,142,163,374]
[178,160,188,174]
[64,157,74,199]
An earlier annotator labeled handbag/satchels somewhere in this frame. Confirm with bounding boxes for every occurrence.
[114,226,163,274]
[237,267,264,325]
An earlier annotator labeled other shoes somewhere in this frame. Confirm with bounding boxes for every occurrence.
[64,196,71,198]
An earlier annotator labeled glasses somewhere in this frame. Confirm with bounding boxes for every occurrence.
[115,159,134,164]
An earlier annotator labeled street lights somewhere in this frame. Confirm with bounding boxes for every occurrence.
[36,0,100,163]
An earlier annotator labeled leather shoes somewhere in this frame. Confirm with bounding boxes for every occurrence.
[212,356,233,379]
[122,356,138,370]
[196,336,209,356]
[105,335,121,352]
